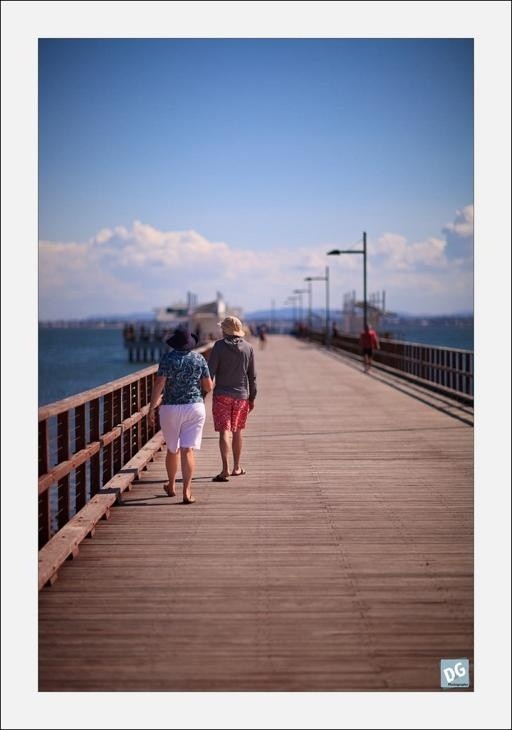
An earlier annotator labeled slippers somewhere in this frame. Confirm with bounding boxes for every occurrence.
[184,496,195,504]
[232,468,246,476]
[212,475,229,482]
[163,483,176,496]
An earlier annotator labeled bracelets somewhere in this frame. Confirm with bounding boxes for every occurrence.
[149,400,158,408]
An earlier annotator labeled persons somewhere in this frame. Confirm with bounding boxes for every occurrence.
[202,315,258,482]
[258,324,267,349]
[360,323,380,372]
[331,321,340,348]
[149,326,213,504]
[293,322,311,342]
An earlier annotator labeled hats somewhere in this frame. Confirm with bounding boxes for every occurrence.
[165,326,198,351]
[217,316,246,336]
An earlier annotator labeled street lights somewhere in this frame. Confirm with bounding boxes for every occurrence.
[283,284,313,328]
[305,265,329,333]
[326,231,368,337]
